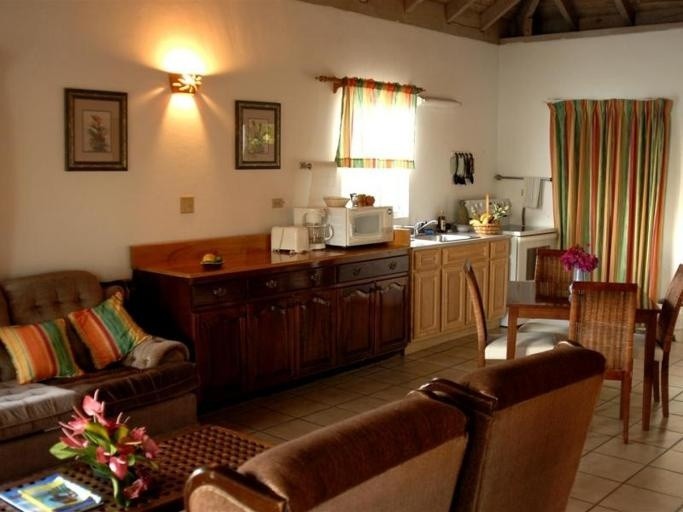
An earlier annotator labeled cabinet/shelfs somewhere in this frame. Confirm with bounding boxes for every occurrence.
[334,248,413,370]
[442,241,490,343]
[135,277,246,409]
[489,238,510,330]
[413,248,442,353]
[246,259,334,394]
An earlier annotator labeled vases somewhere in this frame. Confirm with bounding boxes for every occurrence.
[88,463,116,481]
[570,267,584,283]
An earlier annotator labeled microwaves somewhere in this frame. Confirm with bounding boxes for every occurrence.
[314,206,393,247]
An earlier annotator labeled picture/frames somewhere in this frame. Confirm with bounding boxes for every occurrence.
[235,100,280,168]
[65,89,127,172]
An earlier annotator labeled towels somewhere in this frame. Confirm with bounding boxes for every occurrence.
[522,176,540,208]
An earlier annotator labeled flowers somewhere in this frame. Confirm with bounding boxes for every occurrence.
[49,391,158,506]
[561,244,598,273]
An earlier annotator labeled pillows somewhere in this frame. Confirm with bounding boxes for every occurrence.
[68,293,152,371]
[0,294,87,384]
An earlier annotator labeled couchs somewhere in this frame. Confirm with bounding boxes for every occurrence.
[1,269,201,481]
[182,338,605,512]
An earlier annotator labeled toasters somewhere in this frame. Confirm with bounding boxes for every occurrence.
[270,226,308,254]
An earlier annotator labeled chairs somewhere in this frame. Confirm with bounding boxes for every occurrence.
[533,246,573,284]
[632,264,683,417]
[536,281,570,304]
[465,267,560,367]
[557,282,637,443]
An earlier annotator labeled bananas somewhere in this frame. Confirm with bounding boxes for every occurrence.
[469,213,493,226]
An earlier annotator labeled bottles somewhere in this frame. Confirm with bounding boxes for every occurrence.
[436,210,447,233]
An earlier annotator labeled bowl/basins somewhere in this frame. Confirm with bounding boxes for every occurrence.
[323,197,351,207]
[456,225,471,231]
[200,261,222,267]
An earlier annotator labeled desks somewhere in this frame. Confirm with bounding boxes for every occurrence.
[507,281,660,430]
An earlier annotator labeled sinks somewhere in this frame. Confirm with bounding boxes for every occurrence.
[408,234,481,244]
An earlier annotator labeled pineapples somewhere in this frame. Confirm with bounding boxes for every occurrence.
[492,202,511,224]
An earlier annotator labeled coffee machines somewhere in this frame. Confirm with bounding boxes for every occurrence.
[293,208,334,249]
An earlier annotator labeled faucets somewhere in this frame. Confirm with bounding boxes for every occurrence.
[415,219,438,233]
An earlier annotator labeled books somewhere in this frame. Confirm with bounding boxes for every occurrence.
[0,473,104,512]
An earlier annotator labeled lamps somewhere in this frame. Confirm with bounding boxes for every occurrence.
[144,19,222,95]
